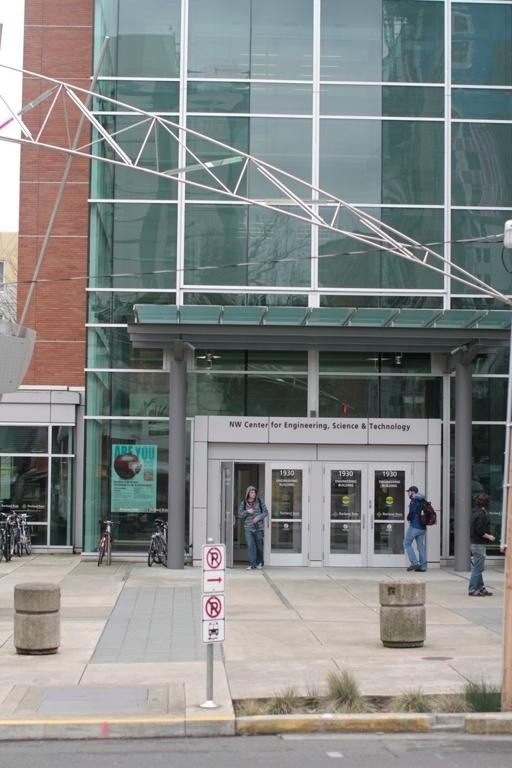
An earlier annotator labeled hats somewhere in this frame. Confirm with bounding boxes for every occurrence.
[406,486,418,493]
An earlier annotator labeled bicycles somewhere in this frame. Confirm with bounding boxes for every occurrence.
[148,518,189,568]
[96,518,121,566]
[0,503,32,561]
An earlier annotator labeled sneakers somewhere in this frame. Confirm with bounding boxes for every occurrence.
[407,564,426,572]
[246,563,263,570]
[469,591,493,596]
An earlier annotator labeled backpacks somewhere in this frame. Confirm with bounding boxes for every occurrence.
[414,499,436,526]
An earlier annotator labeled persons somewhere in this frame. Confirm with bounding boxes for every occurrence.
[469,494,496,596]
[403,486,428,572]
[238,486,268,570]
